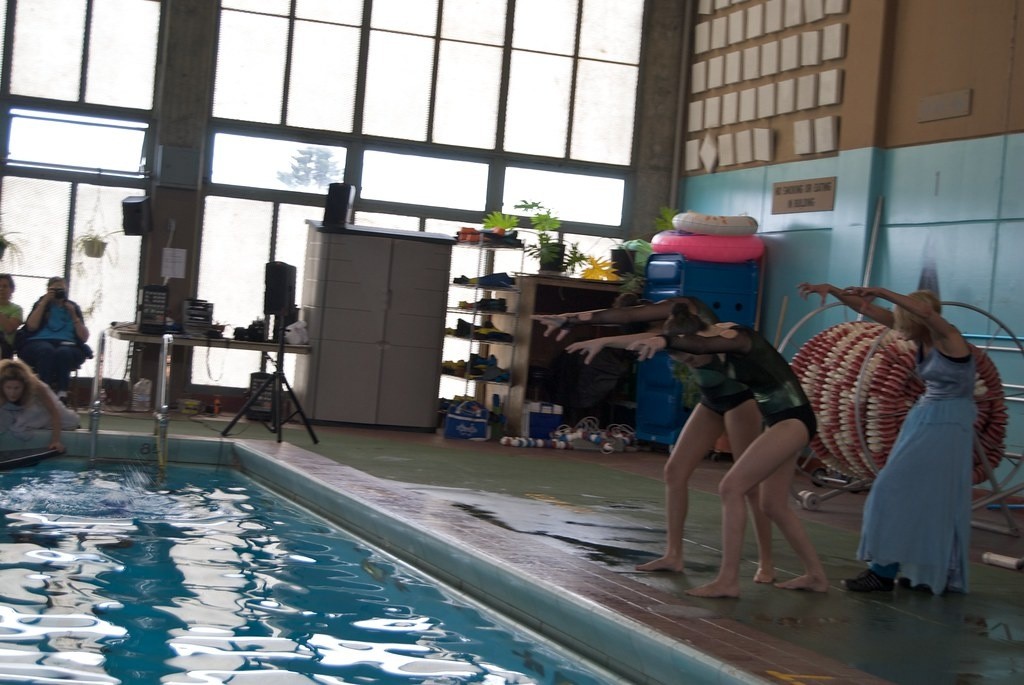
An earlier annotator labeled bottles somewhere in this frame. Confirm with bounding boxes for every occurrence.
[131,378,153,412]
[214,397,221,414]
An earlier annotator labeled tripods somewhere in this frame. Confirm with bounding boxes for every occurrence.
[221,313,319,445]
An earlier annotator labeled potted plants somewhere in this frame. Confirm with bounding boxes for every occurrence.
[481,200,588,274]
[0,212,26,272]
[65,227,125,320]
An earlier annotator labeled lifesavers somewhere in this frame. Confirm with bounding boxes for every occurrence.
[671,209,759,234]
[652,229,764,263]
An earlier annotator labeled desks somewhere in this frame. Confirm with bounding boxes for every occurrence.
[106,323,314,427]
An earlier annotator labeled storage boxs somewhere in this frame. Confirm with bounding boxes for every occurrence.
[526,400,562,441]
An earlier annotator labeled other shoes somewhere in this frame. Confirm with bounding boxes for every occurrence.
[441,226,521,379]
[57,391,68,400]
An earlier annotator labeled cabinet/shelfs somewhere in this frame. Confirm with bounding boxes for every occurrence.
[289,218,457,433]
[433,233,641,443]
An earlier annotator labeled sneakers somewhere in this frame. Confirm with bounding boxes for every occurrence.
[840,569,895,592]
[896,576,931,593]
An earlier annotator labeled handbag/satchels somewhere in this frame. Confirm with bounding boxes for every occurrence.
[443,400,488,439]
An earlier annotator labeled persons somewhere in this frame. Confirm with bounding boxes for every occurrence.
[531,295,829,598]
[0,274,23,359]
[797,282,976,595]
[15,277,89,407]
[0,360,80,452]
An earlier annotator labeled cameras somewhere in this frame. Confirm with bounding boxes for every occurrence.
[48,287,67,299]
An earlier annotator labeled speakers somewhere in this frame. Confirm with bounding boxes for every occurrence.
[122,196,152,235]
[323,183,357,228]
[264,262,296,314]
